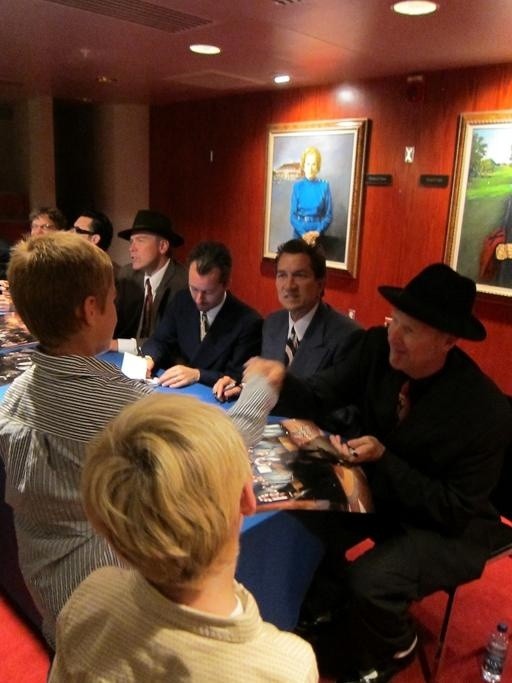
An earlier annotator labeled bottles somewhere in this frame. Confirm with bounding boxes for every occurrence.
[484,622,509,681]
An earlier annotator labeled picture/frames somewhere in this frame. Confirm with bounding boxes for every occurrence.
[263,118,369,279]
[442,109,512,298]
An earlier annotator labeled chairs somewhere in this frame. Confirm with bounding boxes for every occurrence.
[398,393,512,682]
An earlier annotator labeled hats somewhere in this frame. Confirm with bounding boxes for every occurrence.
[118,210,183,248]
[378,263,486,340]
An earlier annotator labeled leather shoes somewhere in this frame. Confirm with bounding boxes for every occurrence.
[335,636,420,683]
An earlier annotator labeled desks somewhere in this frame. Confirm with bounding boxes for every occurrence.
[0,348,354,631]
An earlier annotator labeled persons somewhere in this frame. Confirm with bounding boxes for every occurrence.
[46,391,320,683]
[110,209,189,354]
[212,237,368,420]
[288,146,334,258]
[480,189,512,289]
[0,229,289,663]
[28,206,121,271]
[209,262,512,682]
[138,238,262,389]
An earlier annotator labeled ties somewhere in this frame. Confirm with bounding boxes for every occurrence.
[201,311,208,342]
[140,280,152,337]
[395,383,411,430]
[285,326,301,372]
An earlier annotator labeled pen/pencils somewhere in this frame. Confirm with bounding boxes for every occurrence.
[138,346,150,378]
[213,382,239,396]
[344,440,358,457]
